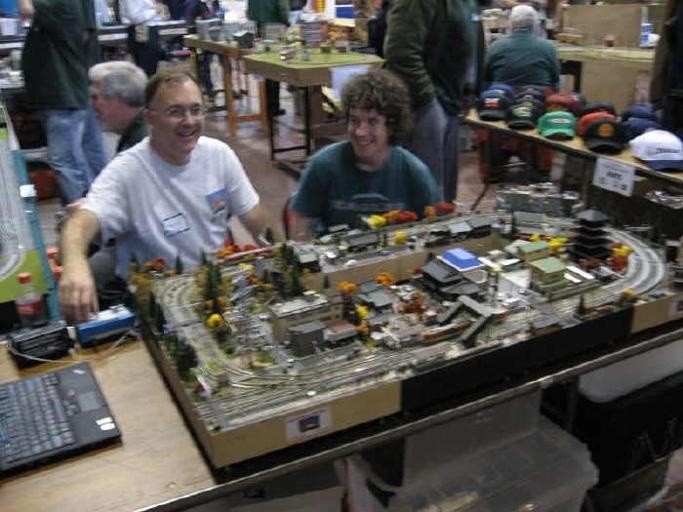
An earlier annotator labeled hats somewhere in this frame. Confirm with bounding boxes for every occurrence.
[478,82,683,171]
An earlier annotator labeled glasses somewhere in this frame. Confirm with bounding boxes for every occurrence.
[165,107,206,120]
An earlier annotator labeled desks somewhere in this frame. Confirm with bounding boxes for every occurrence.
[182,34,386,178]
[0,324,682,510]
[460,105,683,214]
[556,46,656,70]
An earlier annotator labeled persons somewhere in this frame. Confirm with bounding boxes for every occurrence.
[648,0,683,131]
[368,0,560,203]
[287,68,443,242]
[246,0,291,116]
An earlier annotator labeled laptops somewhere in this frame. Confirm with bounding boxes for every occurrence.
[0,362,121,479]
